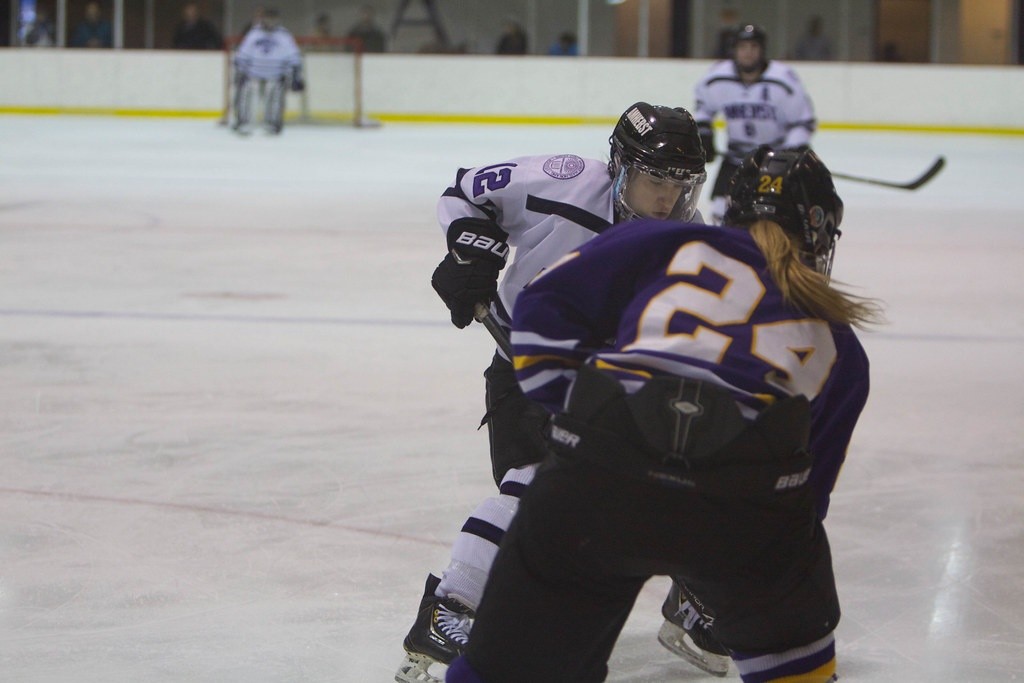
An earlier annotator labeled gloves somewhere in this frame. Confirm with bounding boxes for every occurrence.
[431,216,510,330]
[697,121,714,162]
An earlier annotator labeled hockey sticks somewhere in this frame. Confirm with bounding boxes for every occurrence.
[471,303,736,667]
[712,150,948,191]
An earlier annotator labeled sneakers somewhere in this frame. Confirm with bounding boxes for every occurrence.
[657,581,730,677]
[394,573,475,683]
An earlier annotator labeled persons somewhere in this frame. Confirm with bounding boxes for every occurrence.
[308,14,341,52]
[791,17,834,60]
[345,6,386,51]
[20,7,56,46]
[498,19,527,54]
[232,7,305,134]
[68,3,112,47]
[396,22,871,683]
[170,5,225,50]
[715,9,741,58]
[549,31,578,56]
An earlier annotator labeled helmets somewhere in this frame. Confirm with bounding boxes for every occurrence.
[723,145,843,256]
[731,24,766,50]
[609,102,706,181]
